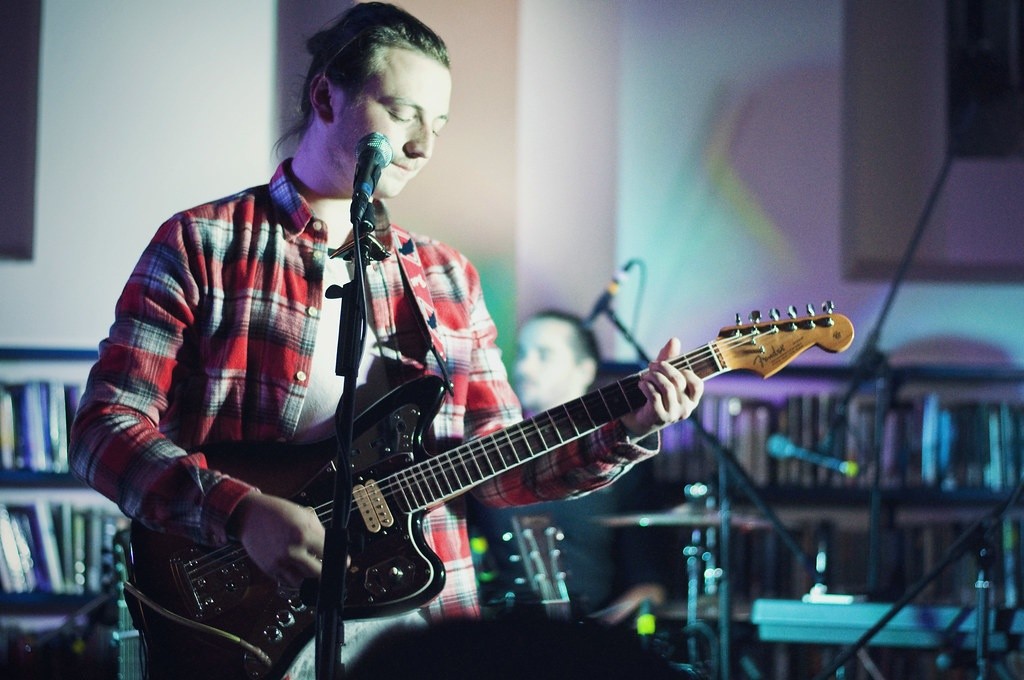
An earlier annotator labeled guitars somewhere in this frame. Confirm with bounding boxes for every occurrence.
[104,515,153,680]
[128,300,855,680]
[499,514,574,622]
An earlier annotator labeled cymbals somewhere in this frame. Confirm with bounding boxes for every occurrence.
[653,590,752,625]
[593,502,774,534]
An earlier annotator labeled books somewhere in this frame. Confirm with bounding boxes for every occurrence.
[730,519,1024,608]
[0,503,132,593]
[704,387,1024,498]
[0,380,83,471]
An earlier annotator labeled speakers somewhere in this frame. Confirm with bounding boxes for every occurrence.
[0,1,41,264]
[944,0,1024,157]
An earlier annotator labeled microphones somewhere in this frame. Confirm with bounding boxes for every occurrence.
[346,128,393,224]
[580,257,635,332]
[761,430,861,480]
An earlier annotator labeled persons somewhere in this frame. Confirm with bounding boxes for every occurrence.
[467,308,677,624]
[66,2,703,680]
